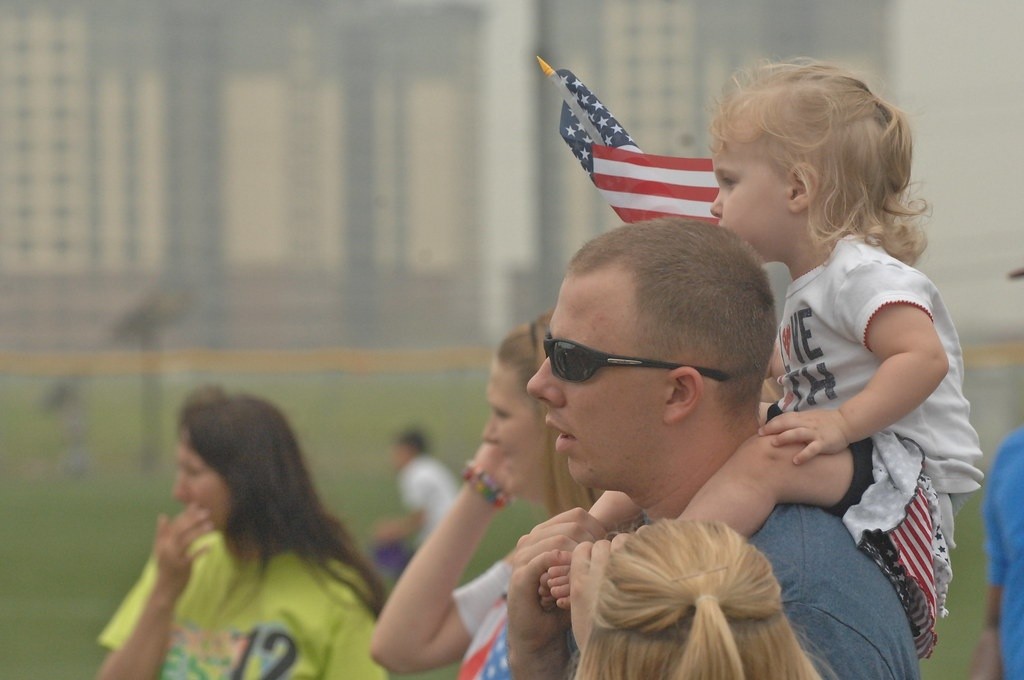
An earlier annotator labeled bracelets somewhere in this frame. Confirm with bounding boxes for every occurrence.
[463,460,509,510]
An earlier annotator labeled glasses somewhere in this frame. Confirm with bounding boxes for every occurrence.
[542,338,732,384]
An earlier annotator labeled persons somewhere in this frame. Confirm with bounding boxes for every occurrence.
[505,216,920,680]
[369,309,604,680]
[367,431,459,578]
[968,269,1024,680]
[573,517,822,680]
[537,56,985,660]
[95,383,391,680]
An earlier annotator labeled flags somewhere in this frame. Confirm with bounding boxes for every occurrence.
[556,69,721,228]
